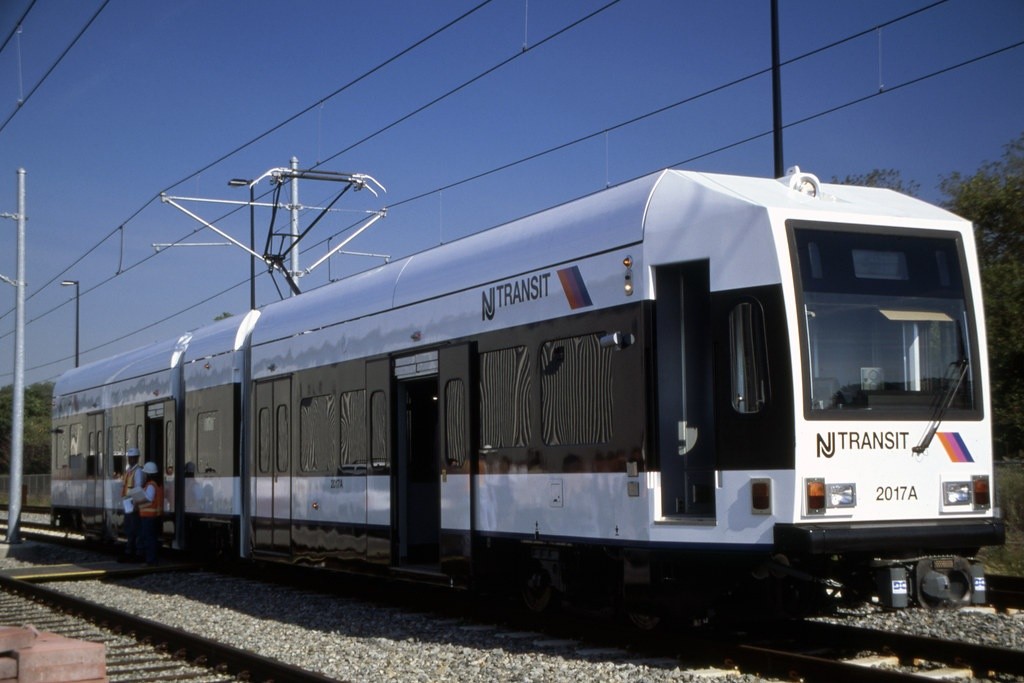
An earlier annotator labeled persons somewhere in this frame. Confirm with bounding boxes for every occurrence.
[122,448,164,566]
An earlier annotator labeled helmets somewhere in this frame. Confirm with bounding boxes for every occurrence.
[141,462,158,473]
[127,448,140,456]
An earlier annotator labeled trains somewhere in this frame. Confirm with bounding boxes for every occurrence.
[48,165,1005,635]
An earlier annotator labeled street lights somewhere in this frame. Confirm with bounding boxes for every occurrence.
[63,281,80,367]
